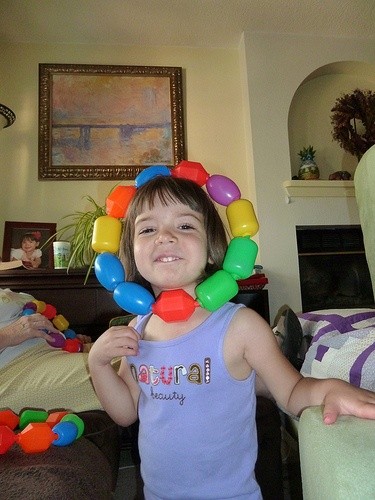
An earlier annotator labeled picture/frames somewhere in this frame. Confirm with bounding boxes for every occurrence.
[2,221,57,270]
[39,61,187,180]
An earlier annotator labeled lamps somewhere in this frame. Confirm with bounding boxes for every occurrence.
[0,104,17,129]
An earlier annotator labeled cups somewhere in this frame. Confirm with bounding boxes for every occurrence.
[52,241,70,269]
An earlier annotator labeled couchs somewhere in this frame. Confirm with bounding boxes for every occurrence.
[279,308,375,500]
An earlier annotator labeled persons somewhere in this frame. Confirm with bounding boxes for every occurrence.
[0,286,308,416]
[11,232,42,267]
[88,175,375,500]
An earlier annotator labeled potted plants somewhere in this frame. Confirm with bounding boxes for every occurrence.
[297,145,320,180]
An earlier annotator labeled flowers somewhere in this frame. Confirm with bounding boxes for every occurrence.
[330,88,375,162]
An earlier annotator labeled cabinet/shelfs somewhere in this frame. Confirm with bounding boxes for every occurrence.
[1,274,269,342]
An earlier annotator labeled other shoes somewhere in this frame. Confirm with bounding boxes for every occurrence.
[272,308,303,369]
